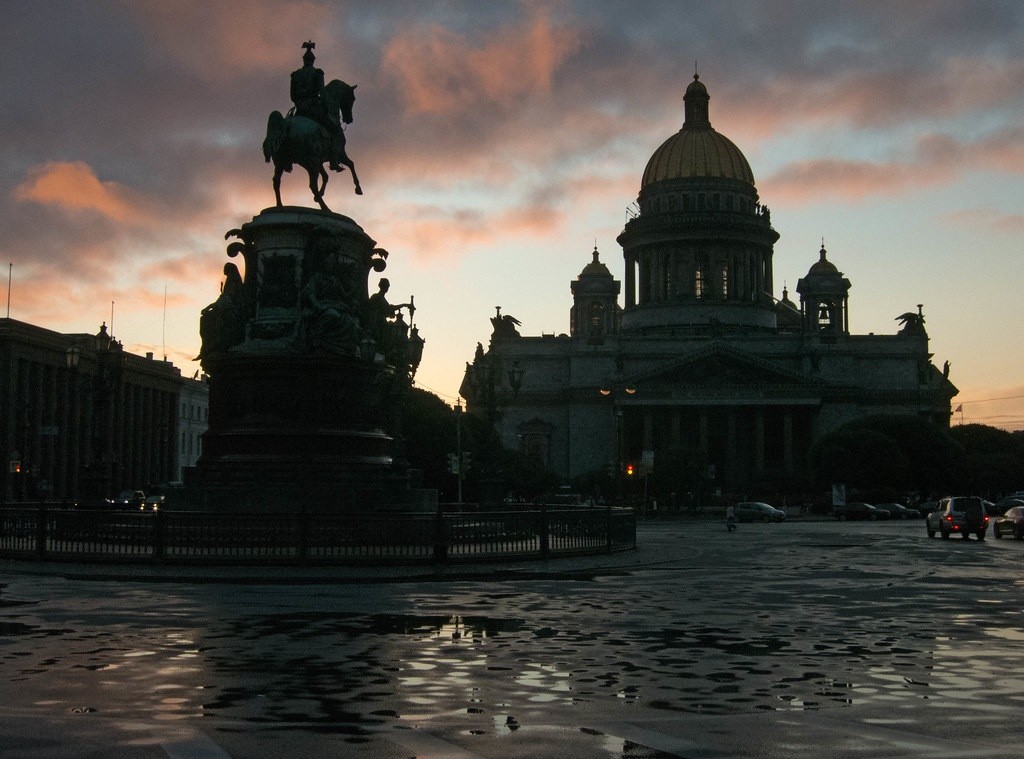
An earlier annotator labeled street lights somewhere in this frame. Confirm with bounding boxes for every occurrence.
[384,310,424,500]
[598,374,638,506]
[65,322,131,500]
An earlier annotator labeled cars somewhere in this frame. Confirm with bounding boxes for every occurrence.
[733,502,787,523]
[833,502,890,522]
[994,506,1024,540]
[141,495,165,512]
[920,490,1024,515]
[104,488,145,510]
[874,503,920,520]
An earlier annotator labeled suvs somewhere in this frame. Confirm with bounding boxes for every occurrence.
[926,496,989,540]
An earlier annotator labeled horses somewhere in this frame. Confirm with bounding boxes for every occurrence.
[261,79,363,212]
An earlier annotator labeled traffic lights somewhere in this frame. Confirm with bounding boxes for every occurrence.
[626,465,634,475]
[462,452,472,470]
[446,452,456,472]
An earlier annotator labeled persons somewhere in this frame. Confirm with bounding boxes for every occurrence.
[191,254,411,361]
[726,501,738,531]
[289,51,346,171]
